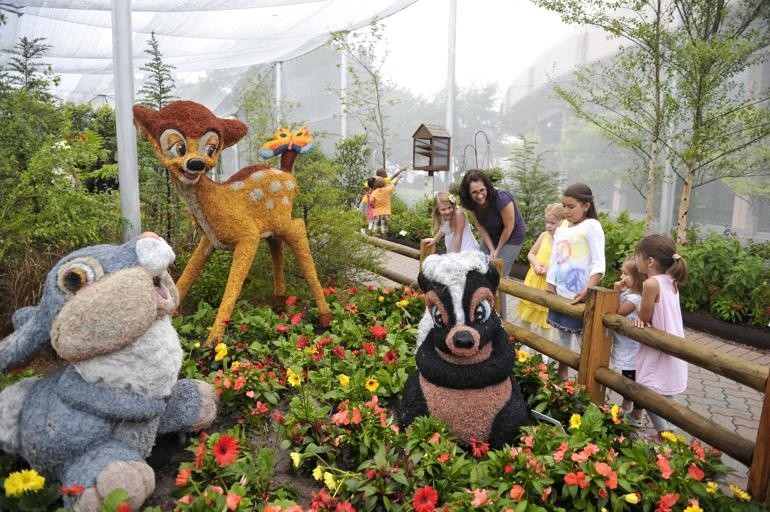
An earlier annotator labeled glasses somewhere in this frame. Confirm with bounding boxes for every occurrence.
[472,189,487,197]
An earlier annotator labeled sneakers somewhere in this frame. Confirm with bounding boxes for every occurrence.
[625,411,642,427]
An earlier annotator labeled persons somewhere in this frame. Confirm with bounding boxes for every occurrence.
[613,254,648,429]
[513,202,567,367]
[633,232,691,445]
[422,191,481,257]
[365,178,377,235]
[370,175,403,238]
[358,166,409,218]
[458,169,528,319]
[543,181,607,381]
